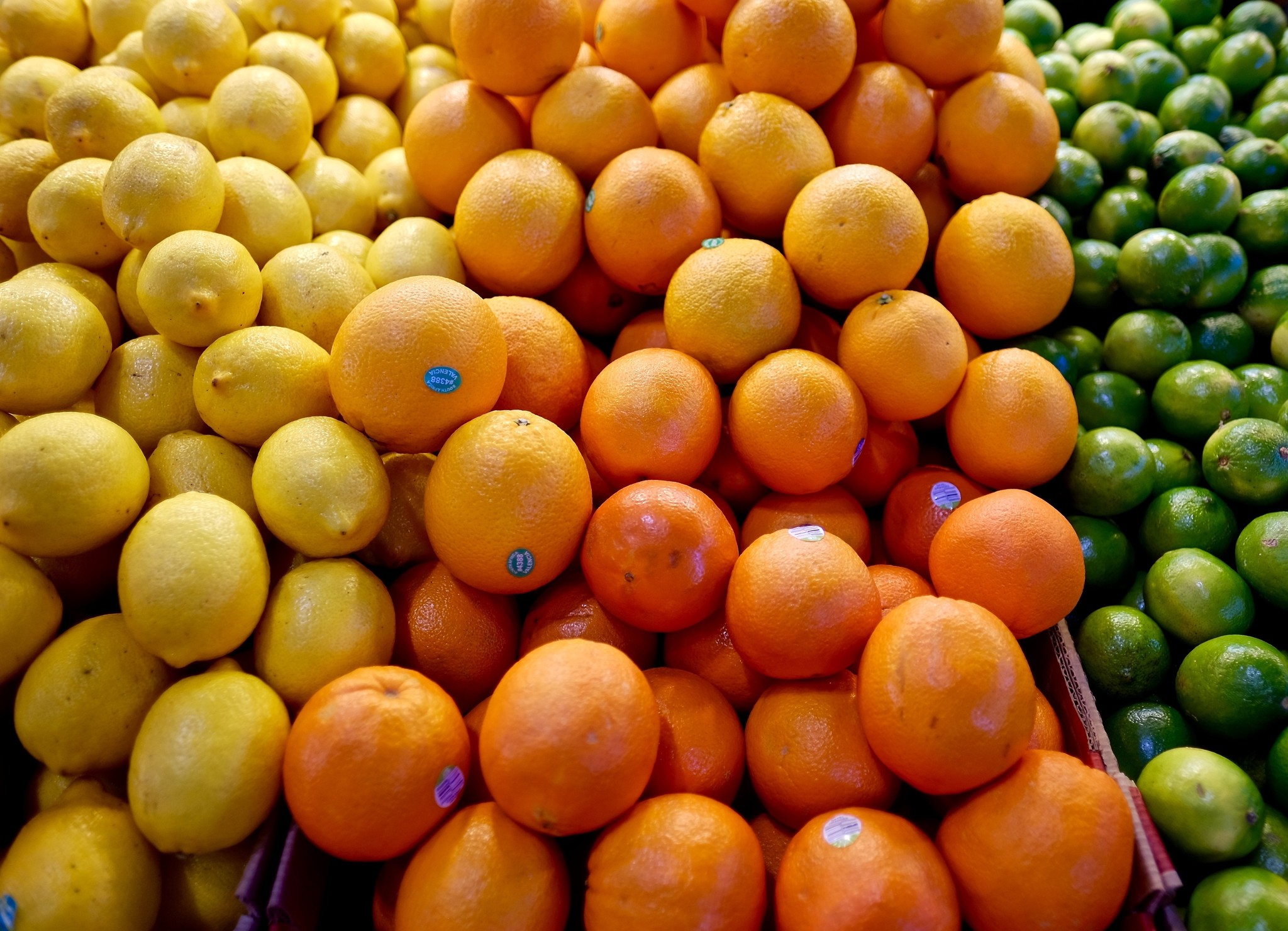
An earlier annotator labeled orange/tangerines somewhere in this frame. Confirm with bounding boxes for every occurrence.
[284,1,1288,931]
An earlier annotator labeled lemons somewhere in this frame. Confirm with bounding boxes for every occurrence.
[1,0,284,931]
